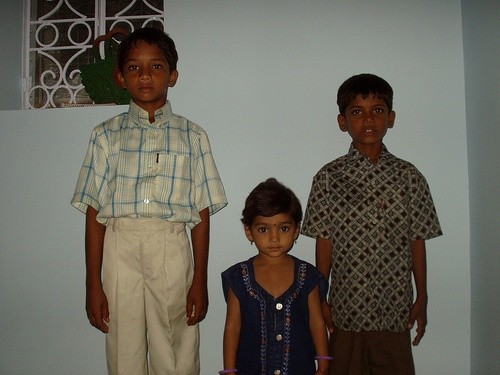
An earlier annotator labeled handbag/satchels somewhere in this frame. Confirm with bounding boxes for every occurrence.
[78,28,132,105]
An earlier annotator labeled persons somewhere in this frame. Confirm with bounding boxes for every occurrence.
[71,27,229,375]
[301,73,442,375]
[218,177,332,375]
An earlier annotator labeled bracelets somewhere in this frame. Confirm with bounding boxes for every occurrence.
[217,368,238,374]
[314,355,333,361]
